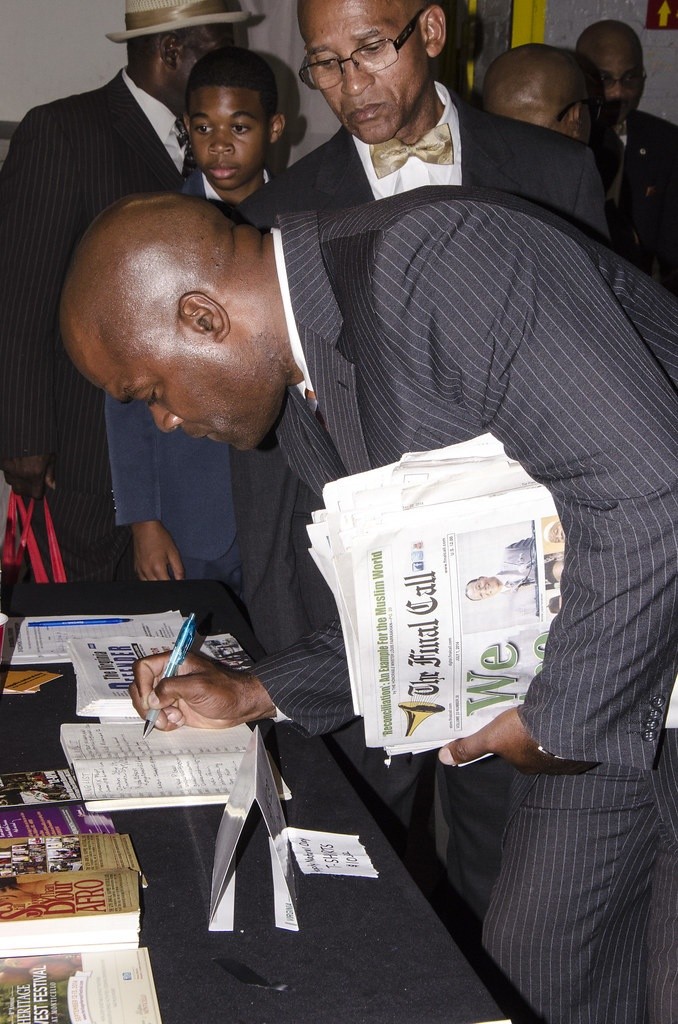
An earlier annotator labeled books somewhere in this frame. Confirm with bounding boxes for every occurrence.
[60,723,291,811]
[66,634,255,717]
[0,768,162,1024]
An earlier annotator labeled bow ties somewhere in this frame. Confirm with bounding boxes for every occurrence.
[369,123,454,179]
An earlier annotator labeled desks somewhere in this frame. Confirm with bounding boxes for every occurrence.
[0,577,508,1024]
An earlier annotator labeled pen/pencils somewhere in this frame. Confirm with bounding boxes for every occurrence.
[142,613,197,737]
[28,618,133,627]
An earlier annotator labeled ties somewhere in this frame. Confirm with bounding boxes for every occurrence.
[304,388,329,434]
[175,116,198,182]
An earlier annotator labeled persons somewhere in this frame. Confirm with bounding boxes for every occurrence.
[482,43,591,144]
[59,184,678,1024]
[545,521,565,543]
[0,0,251,582]
[288,0,612,248]
[176,45,286,209]
[574,19,678,298]
[463,537,537,600]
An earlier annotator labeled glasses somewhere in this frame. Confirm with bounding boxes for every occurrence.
[582,67,647,88]
[299,7,427,91]
[556,94,603,122]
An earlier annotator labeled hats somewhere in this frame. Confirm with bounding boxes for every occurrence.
[105,0,252,44]
